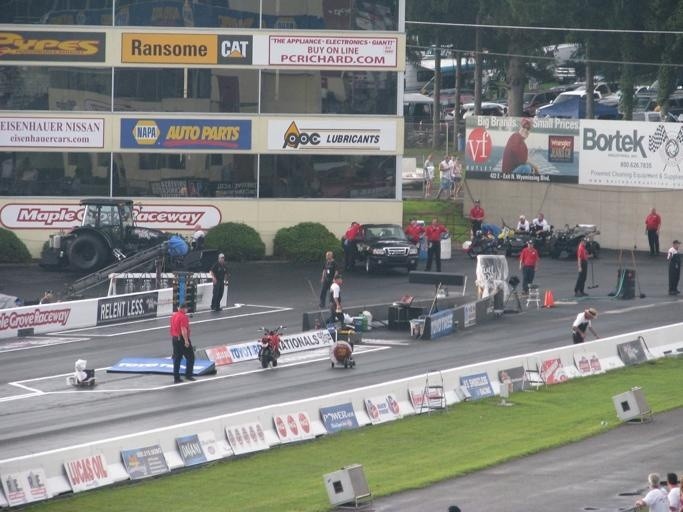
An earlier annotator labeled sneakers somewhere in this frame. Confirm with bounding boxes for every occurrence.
[174,377,196,383]
[669,290,681,295]
[575,291,588,297]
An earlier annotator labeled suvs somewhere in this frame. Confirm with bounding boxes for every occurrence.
[350,222,420,273]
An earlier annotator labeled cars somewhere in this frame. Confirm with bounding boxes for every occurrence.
[401,168,424,189]
[403,42,682,131]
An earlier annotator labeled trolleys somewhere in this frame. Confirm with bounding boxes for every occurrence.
[326,323,356,370]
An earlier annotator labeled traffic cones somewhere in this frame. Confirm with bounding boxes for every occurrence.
[540,287,555,308]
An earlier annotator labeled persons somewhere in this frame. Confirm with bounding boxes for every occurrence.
[208,253,232,312]
[189,223,204,244]
[340,222,362,271]
[664,472,681,512]
[38,288,53,305]
[468,199,551,255]
[422,217,448,273]
[316,250,338,307]
[501,117,540,176]
[422,151,464,201]
[328,273,344,328]
[518,239,540,295]
[642,207,662,258]
[572,237,590,297]
[571,307,600,344]
[168,302,198,384]
[634,470,670,512]
[401,216,426,253]
[666,240,681,295]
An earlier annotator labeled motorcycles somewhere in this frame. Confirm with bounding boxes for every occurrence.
[253,323,286,369]
[460,221,603,261]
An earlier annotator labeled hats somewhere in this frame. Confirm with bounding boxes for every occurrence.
[585,309,597,318]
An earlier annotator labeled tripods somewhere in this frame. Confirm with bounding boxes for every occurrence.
[505,288,523,313]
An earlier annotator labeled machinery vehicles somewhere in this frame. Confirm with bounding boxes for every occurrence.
[34,194,171,272]
